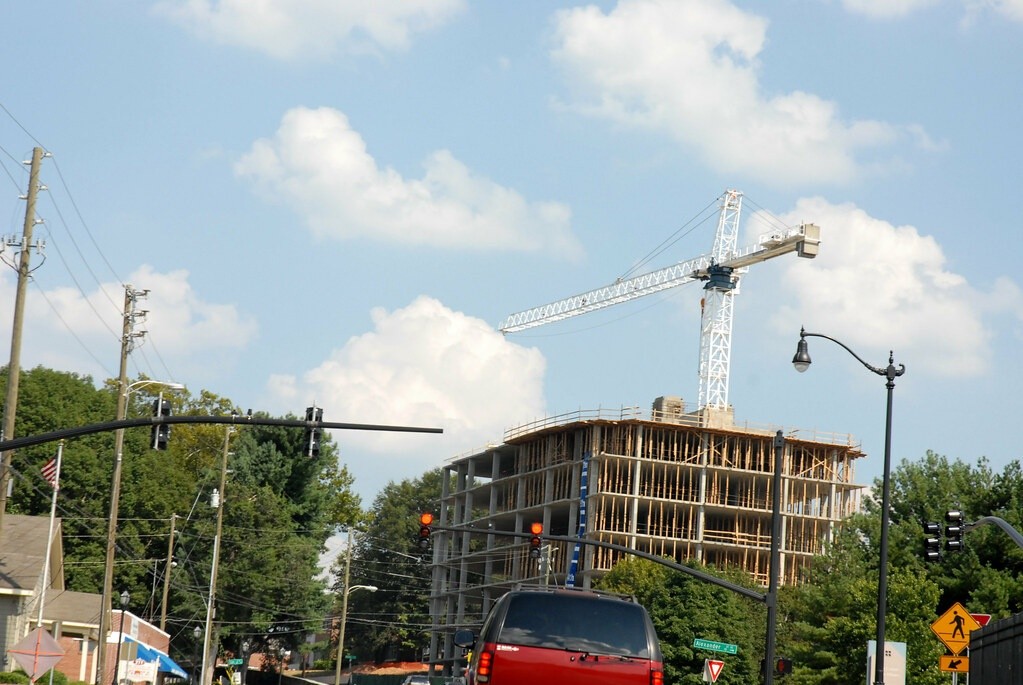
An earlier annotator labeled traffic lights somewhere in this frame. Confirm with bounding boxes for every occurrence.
[774,658,790,674]
[924,522,941,562]
[418,513,433,551]
[944,511,963,552]
[530,523,542,558]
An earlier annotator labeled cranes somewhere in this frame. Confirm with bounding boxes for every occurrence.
[495,188,821,425]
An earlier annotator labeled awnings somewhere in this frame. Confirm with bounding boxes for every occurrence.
[125,635,188,679]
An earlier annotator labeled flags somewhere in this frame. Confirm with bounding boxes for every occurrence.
[41,449,60,492]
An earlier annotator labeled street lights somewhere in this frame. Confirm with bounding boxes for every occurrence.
[111,590,131,685]
[334,585,378,685]
[792,325,907,685]
[94,381,185,685]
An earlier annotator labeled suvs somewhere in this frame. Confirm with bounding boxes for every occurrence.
[453,582,664,685]
